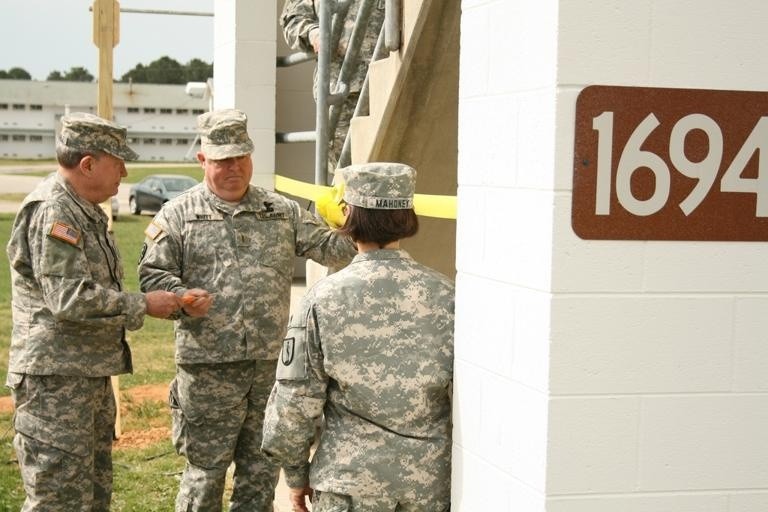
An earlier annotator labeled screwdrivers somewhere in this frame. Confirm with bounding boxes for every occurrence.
[178,292,216,302]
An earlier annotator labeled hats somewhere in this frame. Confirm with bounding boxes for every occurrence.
[57,110,142,164]
[336,159,418,213]
[195,108,255,161]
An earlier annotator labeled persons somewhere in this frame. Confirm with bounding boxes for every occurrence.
[258,161,455,512]
[137,109,356,512]
[278,0,401,177]
[5,110,182,511]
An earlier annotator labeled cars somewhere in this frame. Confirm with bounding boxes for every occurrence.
[126,173,202,217]
[35,169,121,221]
